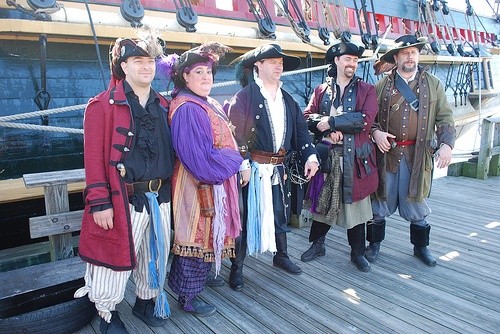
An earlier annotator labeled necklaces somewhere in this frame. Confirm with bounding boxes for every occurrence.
[398,74,416,80]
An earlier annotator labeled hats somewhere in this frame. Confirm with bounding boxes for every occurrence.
[380,34,426,64]
[156,42,233,81]
[325,43,365,63]
[226,44,301,87]
[116,25,166,63]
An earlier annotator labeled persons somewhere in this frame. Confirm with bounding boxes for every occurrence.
[75,38,173,334]
[364,34,456,266]
[168,52,242,317]
[301,42,379,272]
[227,44,321,292]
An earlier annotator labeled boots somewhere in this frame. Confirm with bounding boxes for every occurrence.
[410,222,437,267]
[229,251,246,292]
[351,245,371,272]
[132,297,166,327]
[273,233,302,275]
[100,311,130,334]
[364,220,385,263]
[301,236,326,262]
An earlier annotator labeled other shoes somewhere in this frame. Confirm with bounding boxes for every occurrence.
[178,292,217,317]
[205,274,224,286]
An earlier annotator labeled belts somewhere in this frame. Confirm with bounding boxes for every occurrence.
[250,153,286,165]
[396,141,416,146]
[126,178,165,194]
